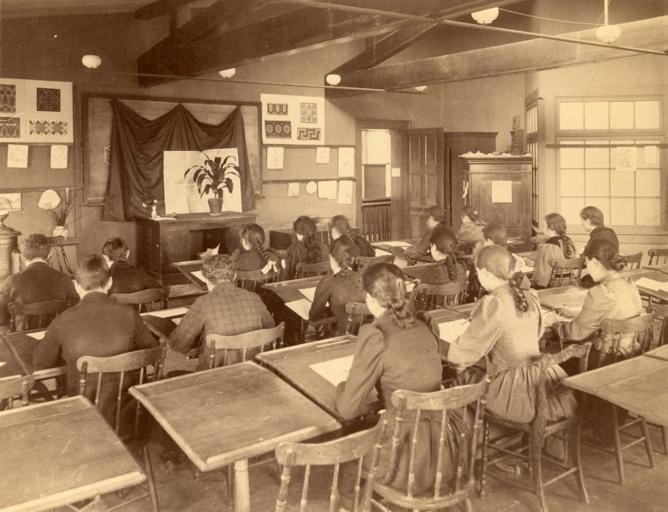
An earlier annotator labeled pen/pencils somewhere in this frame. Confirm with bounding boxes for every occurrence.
[461,317,470,325]
[315,339,350,348]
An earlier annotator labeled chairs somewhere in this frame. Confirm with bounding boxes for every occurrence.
[368,381,489,512]
[273,407,385,510]
[577,309,655,482]
[472,339,592,512]
[79,339,165,511]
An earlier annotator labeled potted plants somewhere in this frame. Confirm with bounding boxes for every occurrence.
[183,146,242,216]
[42,192,79,242]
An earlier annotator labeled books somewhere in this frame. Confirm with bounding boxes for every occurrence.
[635,275,668,303]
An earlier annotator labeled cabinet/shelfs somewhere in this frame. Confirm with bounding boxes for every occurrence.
[137,210,257,283]
[461,157,533,238]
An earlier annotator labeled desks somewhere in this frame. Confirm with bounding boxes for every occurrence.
[287,239,667,360]
[127,360,341,509]
[254,331,383,417]
[2,394,148,511]
[565,355,667,428]
[0,238,284,403]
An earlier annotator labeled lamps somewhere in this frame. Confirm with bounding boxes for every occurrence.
[82,54,103,71]
[471,6,499,25]
[595,0,622,43]
[326,74,342,88]
[219,67,238,82]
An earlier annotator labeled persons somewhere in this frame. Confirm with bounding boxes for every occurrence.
[1,206,643,511]
